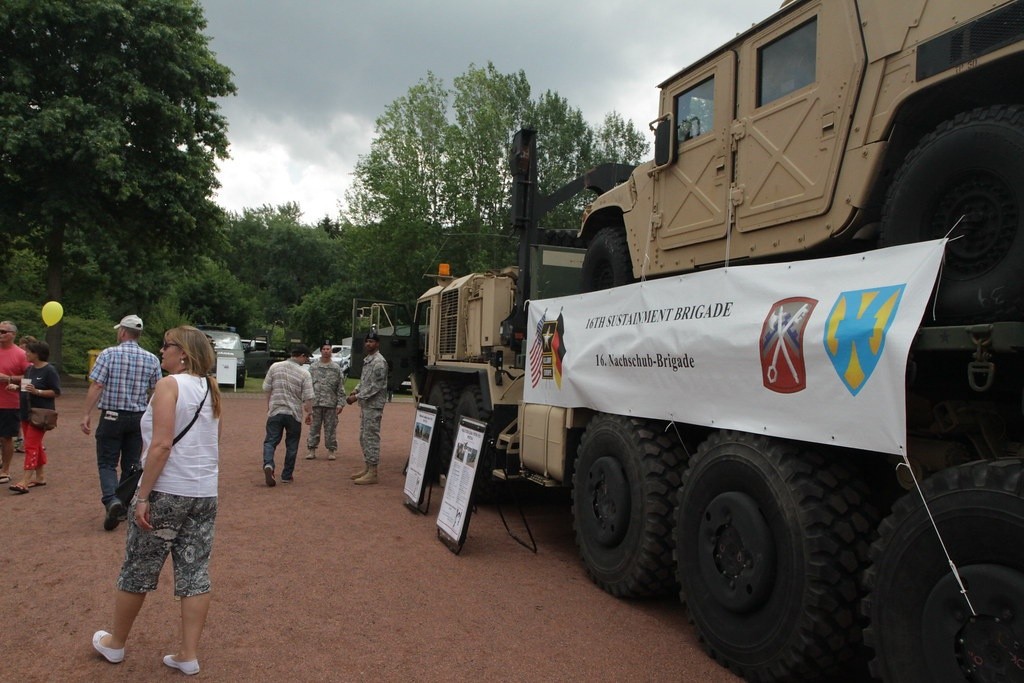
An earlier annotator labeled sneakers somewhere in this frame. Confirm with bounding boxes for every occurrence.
[281,476,293,482]
[92,629,124,663]
[264,466,276,487]
[103,497,128,530]
[163,655,200,675]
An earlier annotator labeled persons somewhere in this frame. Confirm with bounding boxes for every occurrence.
[262,344,316,486]
[0,321,61,492]
[92,324,224,683]
[306,339,346,460]
[346,333,388,485]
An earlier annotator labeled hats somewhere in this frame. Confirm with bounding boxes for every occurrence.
[114,314,143,330]
[365,333,379,341]
[320,338,332,350]
[292,343,314,358]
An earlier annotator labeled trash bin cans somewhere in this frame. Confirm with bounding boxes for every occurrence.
[87,348,103,383]
[224,359,246,388]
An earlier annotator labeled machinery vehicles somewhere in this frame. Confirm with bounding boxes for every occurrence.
[347,126,1024,683]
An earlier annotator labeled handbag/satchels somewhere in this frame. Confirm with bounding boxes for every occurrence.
[114,469,143,506]
[29,408,58,431]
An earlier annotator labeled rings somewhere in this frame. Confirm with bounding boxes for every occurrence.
[138,499,148,503]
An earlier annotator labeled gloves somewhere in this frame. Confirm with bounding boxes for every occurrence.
[349,388,358,396]
[345,394,357,405]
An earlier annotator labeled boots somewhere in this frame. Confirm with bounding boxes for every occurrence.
[355,465,378,484]
[350,464,368,479]
[305,447,315,459]
[328,448,336,460]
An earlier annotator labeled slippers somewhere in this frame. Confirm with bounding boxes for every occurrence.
[9,482,30,494]
[29,481,47,488]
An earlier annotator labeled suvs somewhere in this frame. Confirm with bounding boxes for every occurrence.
[308,345,349,359]
[189,324,253,389]
[571,0,1024,289]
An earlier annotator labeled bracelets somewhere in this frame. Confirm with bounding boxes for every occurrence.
[309,412,314,414]
[38,390,41,395]
[10,376,13,382]
[81,315,163,531]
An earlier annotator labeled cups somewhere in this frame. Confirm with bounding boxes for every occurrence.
[20,378,32,391]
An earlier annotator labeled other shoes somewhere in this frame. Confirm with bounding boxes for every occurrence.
[14,438,24,452]
[0,472,12,482]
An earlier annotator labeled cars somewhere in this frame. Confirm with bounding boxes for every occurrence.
[329,349,352,372]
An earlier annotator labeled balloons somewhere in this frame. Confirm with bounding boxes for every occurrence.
[42,301,63,327]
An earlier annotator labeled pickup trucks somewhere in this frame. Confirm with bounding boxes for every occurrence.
[240,339,287,379]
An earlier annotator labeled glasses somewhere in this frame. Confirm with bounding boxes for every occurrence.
[0,330,14,334]
[163,338,178,350]
